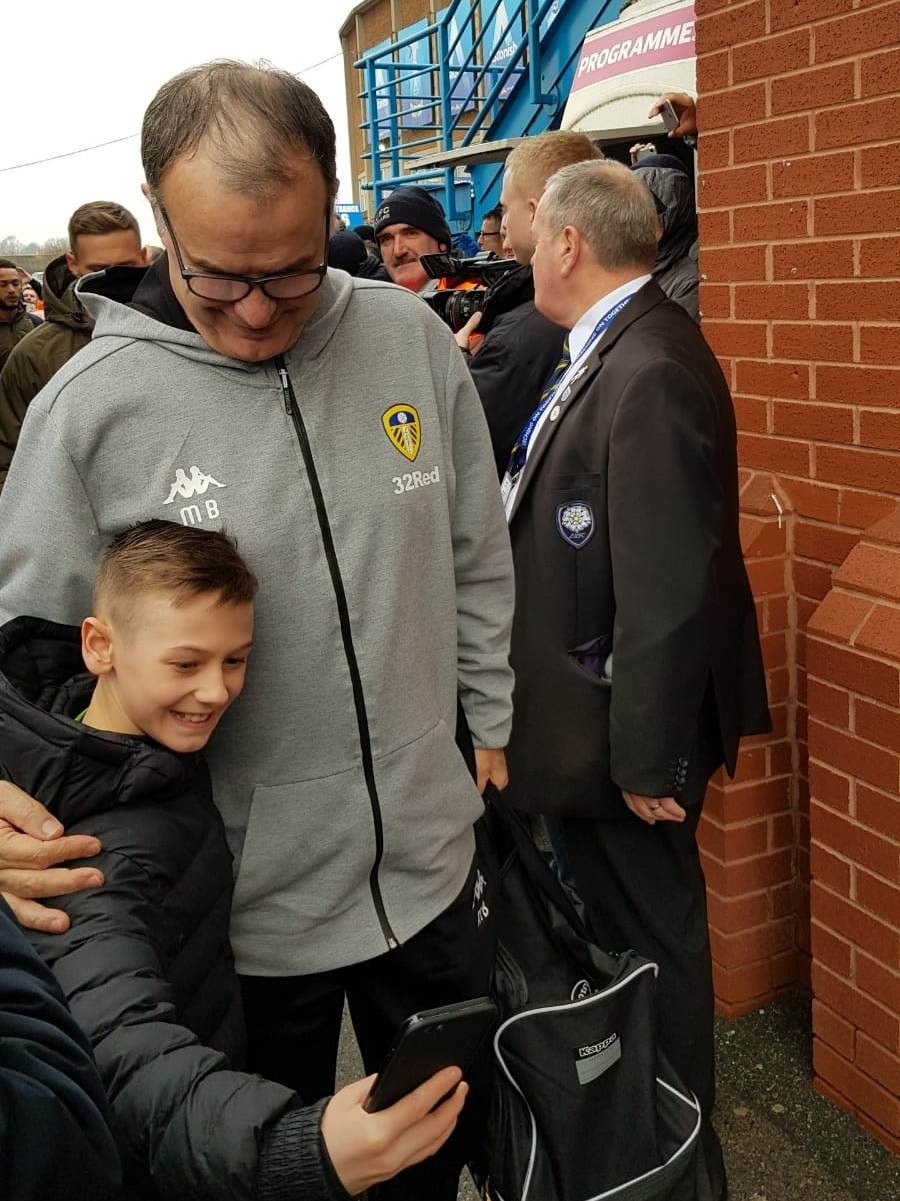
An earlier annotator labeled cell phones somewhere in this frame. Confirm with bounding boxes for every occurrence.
[658,99,679,131]
[634,145,659,163]
[366,997,500,1116]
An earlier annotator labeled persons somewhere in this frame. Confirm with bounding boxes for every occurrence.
[0,62,517,1200]
[0,92,697,490]
[499,160,775,1127]
[0,520,467,1201]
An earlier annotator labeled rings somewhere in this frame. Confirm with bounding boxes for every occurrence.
[649,803,661,811]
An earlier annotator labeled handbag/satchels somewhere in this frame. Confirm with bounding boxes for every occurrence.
[471,784,732,1201]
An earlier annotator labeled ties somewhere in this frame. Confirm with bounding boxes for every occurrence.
[507,332,571,478]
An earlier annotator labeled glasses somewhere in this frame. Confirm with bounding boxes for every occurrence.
[154,195,332,303]
[480,230,500,236]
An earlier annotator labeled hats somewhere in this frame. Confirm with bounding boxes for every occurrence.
[327,186,452,270]
